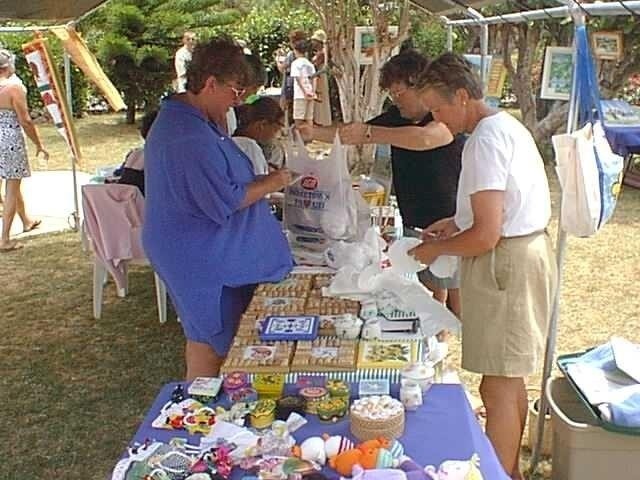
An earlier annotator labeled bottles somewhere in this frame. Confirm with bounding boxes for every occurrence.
[400,379,422,411]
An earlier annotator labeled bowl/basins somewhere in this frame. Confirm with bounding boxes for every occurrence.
[402,364,436,393]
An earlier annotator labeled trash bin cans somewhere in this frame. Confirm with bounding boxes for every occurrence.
[546,377,640,480]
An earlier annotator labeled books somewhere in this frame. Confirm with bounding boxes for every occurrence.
[221,270,416,373]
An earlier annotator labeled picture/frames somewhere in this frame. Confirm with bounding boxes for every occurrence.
[540,46,573,101]
[591,31,626,62]
[354,26,399,64]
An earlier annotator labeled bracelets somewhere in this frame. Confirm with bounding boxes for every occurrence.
[365,124,372,143]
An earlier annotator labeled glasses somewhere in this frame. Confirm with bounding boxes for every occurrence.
[210,71,248,101]
[387,87,411,102]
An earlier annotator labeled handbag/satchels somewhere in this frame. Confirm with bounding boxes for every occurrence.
[551,121,624,238]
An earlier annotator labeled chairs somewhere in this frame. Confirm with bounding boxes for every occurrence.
[81,184,168,324]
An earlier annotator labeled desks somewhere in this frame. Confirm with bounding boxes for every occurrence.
[113,383,503,480]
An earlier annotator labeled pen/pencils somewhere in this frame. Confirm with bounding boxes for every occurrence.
[268,161,279,171]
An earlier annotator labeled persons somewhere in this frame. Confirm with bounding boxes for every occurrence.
[407,52,558,480]
[290,50,467,318]
[106,110,158,199]
[229,97,285,216]
[278,30,333,128]
[175,31,195,93]
[0,49,48,252]
[226,54,267,138]
[140,31,296,384]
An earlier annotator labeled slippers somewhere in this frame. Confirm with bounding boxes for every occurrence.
[0,239,23,252]
[23,219,42,232]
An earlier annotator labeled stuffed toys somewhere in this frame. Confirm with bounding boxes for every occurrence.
[292,433,356,463]
[330,445,398,474]
[339,453,483,480]
[355,437,405,459]
[240,420,302,480]
